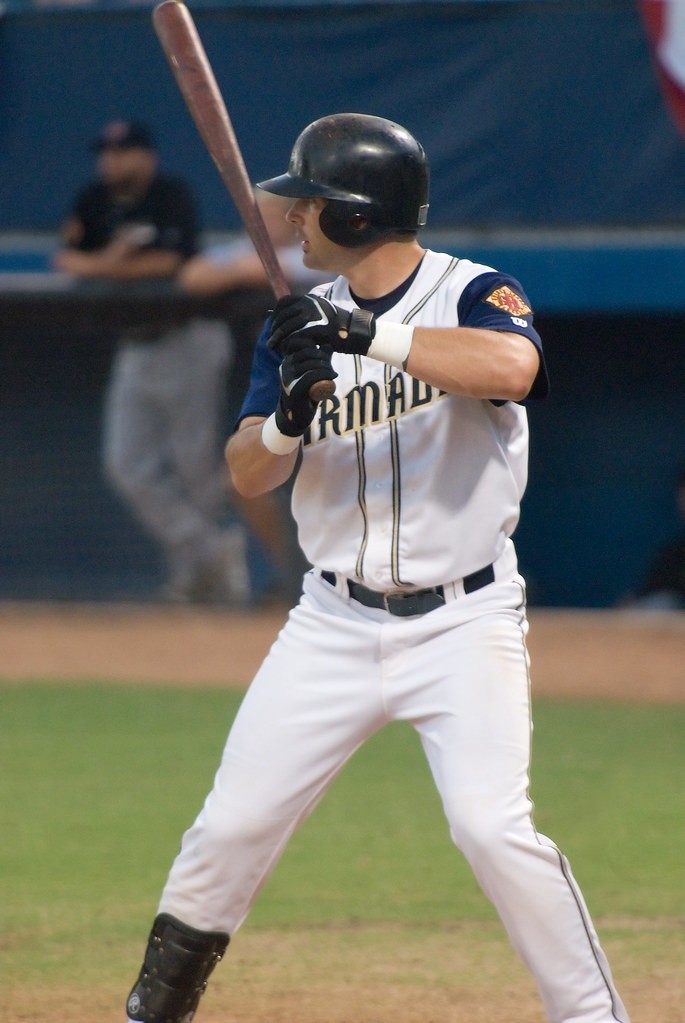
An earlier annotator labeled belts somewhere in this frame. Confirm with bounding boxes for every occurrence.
[322,563,495,617]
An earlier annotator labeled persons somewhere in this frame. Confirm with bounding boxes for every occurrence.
[177,186,311,590]
[125,113,630,1022]
[54,118,253,603]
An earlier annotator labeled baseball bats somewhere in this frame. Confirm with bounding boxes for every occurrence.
[150,0,337,402]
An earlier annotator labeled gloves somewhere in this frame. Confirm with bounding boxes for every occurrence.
[264,292,375,357]
[272,345,338,437]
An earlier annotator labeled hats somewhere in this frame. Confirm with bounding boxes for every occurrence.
[90,121,152,146]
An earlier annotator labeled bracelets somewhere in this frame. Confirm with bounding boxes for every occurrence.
[262,412,303,455]
[365,320,413,372]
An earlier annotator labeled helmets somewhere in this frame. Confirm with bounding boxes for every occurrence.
[257,112,431,248]
